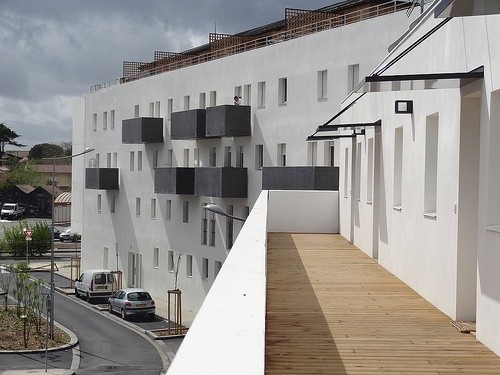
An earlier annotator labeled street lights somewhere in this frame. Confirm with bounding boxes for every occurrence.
[42,146,96,339]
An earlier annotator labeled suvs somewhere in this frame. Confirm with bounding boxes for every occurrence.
[73,269,117,303]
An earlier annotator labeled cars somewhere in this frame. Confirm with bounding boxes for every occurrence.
[49,226,63,240]
[59,228,82,242]
[108,287,155,321]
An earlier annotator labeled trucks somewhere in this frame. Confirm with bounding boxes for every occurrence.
[0,203,25,220]
